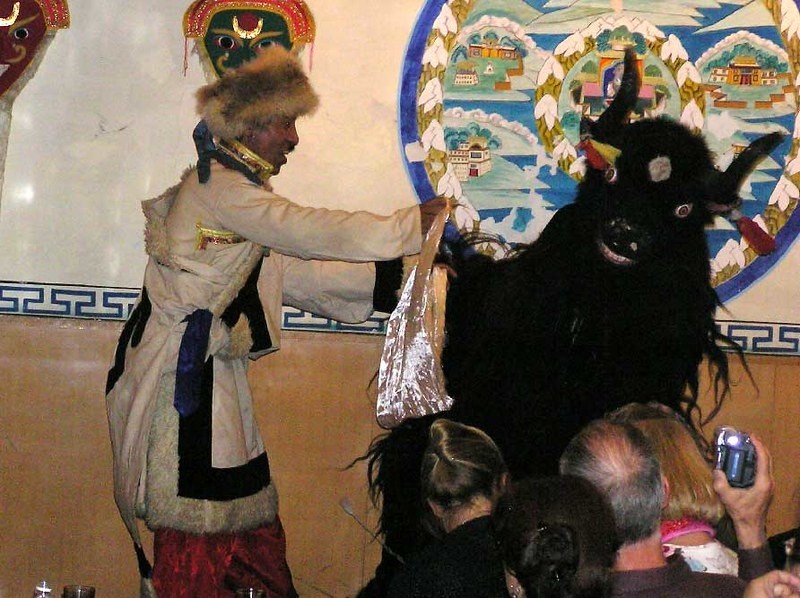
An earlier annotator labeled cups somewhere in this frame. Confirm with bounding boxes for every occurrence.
[234,587,267,598]
[63,585,96,598]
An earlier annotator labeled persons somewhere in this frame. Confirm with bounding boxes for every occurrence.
[102,49,458,598]
[352,400,800,598]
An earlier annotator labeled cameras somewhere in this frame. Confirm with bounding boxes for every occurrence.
[712,427,757,488]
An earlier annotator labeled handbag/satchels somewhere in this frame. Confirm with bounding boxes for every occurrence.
[372,203,452,430]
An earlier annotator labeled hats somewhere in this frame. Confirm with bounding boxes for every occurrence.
[195,48,318,142]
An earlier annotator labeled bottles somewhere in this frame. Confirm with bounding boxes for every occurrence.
[30,580,54,598]
